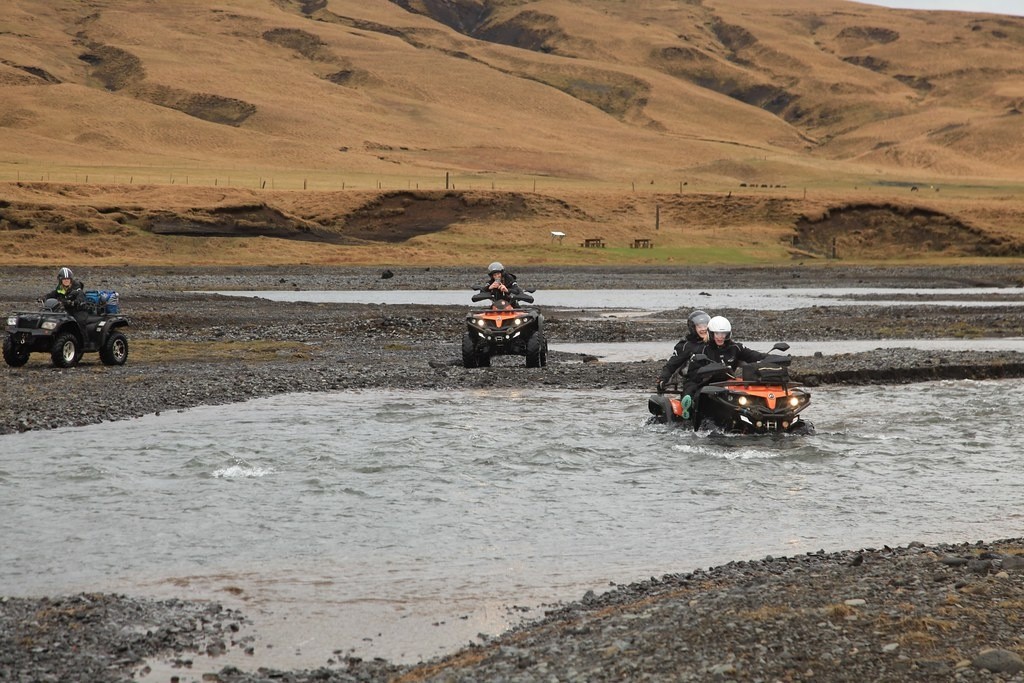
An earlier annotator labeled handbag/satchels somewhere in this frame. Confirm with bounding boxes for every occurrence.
[100,290,120,313]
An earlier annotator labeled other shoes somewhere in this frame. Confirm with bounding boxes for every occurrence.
[84,338,90,347]
[681,395,692,420]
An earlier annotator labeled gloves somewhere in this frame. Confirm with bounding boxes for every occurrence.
[36,296,47,303]
[655,379,666,393]
[700,363,721,377]
[73,300,81,307]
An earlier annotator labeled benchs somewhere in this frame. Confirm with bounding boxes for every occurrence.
[629,243,653,248]
[579,243,607,248]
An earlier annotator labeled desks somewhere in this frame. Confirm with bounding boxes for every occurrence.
[583,239,605,247]
[635,239,651,248]
[551,232,565,245]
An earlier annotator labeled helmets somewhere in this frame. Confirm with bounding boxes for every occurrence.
[57,268,74,285]
[487,262,504,275]
[706,316,732,342]
[687,310,712,339]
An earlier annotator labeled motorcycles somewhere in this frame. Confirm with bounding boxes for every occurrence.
[647,343,814,438]
[1,290,131,368]
[461,284,548,369]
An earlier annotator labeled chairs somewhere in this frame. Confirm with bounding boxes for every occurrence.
[85,291,100,306]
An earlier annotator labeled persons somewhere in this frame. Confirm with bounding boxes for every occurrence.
[37,267,86,314]
[655,309,711,394]
[481,262,520,308]
[679,314,772,420]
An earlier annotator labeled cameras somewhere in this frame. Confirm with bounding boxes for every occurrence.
[495,278,500,282]
